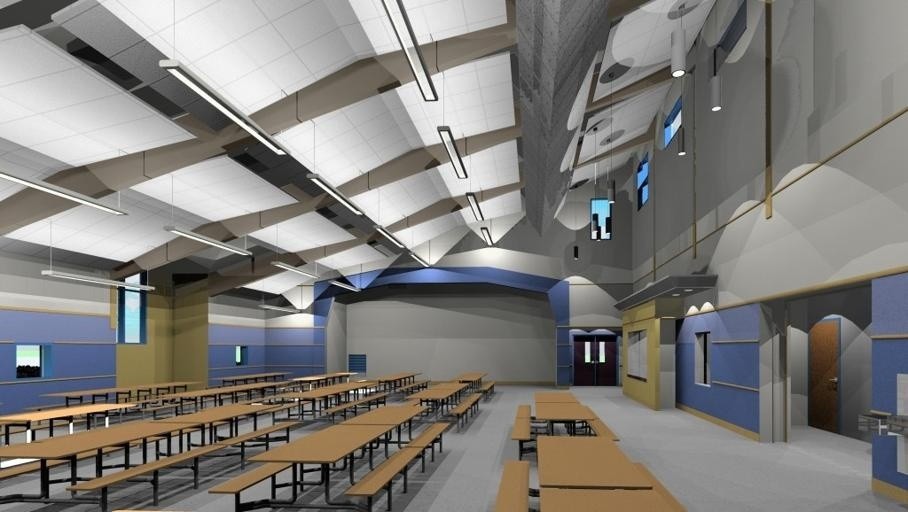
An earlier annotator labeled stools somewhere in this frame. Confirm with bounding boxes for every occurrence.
[495,389,686,511]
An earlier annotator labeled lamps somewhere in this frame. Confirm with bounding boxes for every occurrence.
[709,44,724,113]
[585,71,625,235]
[668,0,689,157]
